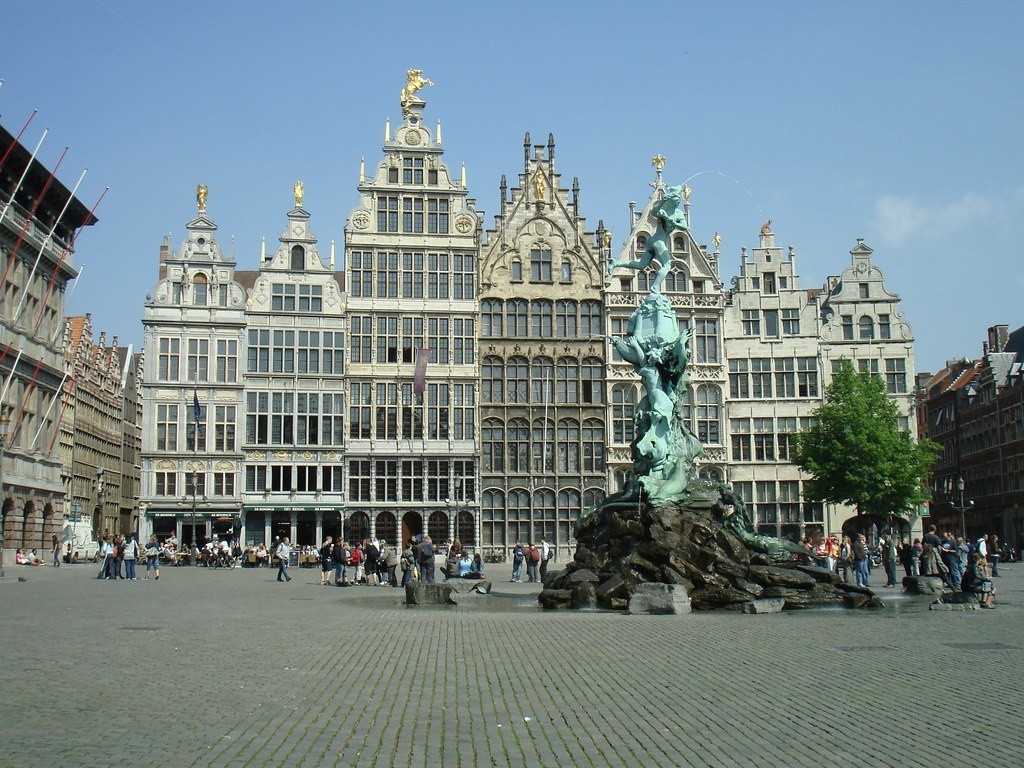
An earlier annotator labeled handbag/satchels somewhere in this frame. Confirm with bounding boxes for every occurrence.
[145,542,159,556]
[133,540,137,556]
[113,546,117,557]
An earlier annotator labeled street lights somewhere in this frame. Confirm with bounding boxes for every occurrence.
[190,470,199,567]
[453,473,463,540]
[957,476,966,543]
[98,488,106,558]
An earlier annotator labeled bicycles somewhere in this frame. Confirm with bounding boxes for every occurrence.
[207,548,236,570]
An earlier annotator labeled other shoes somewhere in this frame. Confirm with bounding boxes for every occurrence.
[516,579,523,583]
[141,577,149,580]
[992,574,1001,577]
[155,577,160,580]
[286,577,292,582]
[980,603,997,609]
[512,579,516,581]
[277,578,284,582]
[320,579,397,587]
[882,584,895,588]
[106,576,136,580]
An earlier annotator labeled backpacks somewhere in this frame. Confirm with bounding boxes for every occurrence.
[272,544,283,559]
[351,549,360,565]
[545,544,554,559]
[528,547,539,562]
[422,542,432,558]
[400,553,409,571]
[515,548,524,563]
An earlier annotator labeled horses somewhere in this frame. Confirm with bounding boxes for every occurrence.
[400,70,434,102]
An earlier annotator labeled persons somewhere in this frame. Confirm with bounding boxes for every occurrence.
[510,537,553,583]
[535,171,547,200]
[712,232,721,252]
[196,184,208,212]
[607,184,688,295]
[276,536,291,582]
[797,523,1018,609]
[293,180,304,206]
[320,535,438,588]
[97,529,319,580]
[16,535,62,567]
[603,228,612,249]
[440,539,486,580]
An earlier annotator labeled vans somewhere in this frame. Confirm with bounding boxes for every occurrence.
[69,540,101,563]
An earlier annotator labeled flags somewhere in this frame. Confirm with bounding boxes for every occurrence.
[413,347,430,394]
